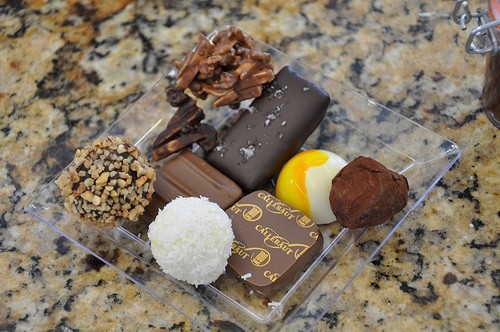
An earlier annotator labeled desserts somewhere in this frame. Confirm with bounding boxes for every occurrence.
[224,189,324,295]
[58,136,155,228]
[152,75,218,162]
[153,150,243,209]
[328,156,409,230]
[275,149,348,225]
[148,194,236,288]
[174,28,275,110]
[207,65,332,193]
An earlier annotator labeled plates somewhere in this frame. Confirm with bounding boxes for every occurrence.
[22,23,464,332]
